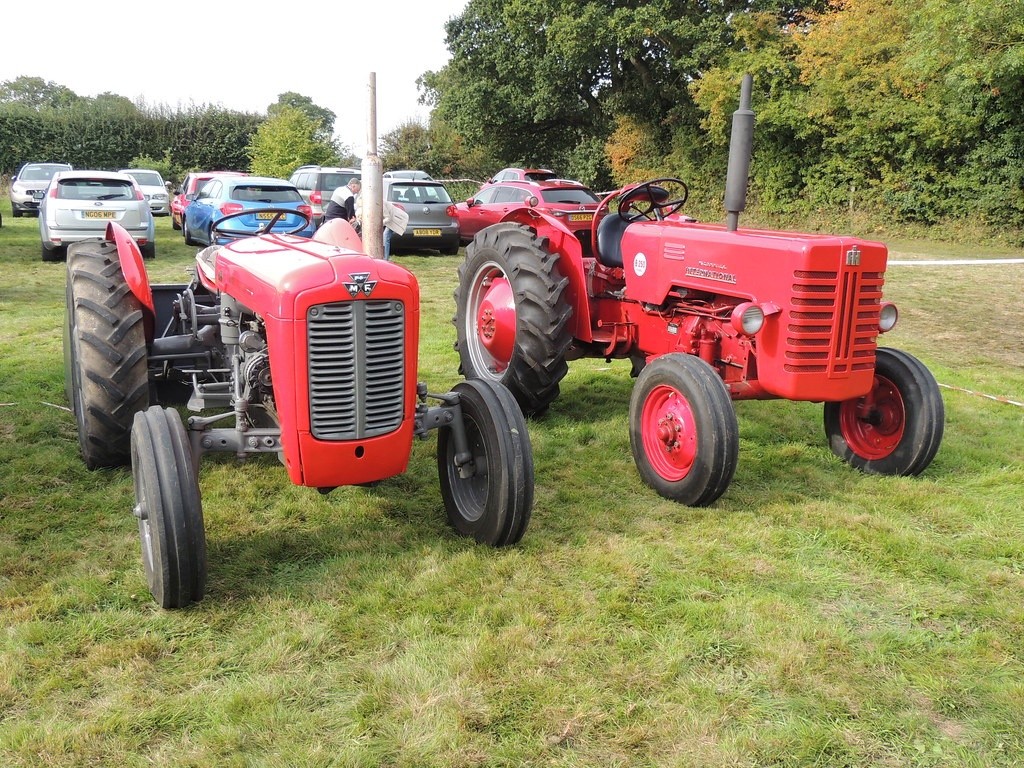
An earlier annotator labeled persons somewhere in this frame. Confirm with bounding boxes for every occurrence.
[324,177,361,233]
[355,198,409,260]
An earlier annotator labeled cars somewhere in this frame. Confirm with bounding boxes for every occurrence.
[172,171,249,231]
[455,179,609,247]
[118,169,173,217]
[480,168,557,193]
[32,171,155,260]
[380,170,438,182]
[180,177,317,246]
[9,162,74,218]
[288,164,362,223]
[382,177,461,256]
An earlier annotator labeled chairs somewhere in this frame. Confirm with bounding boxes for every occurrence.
[404,189,416,198]
[64,183,80,199]
[393,191,401,200]
[592,184,670,268]
[109,187,123,194]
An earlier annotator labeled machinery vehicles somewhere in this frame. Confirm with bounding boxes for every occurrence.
[451,177,947,507]
[64,207,537,610]
